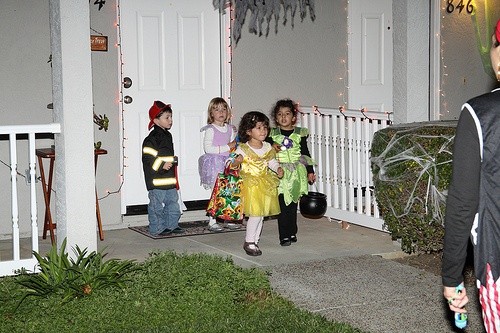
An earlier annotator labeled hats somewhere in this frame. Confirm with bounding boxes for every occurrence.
[146,100,172,131]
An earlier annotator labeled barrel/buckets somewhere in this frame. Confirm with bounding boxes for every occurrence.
[300,191,327,219]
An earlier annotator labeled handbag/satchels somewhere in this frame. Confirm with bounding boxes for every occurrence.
[206,158,244,221]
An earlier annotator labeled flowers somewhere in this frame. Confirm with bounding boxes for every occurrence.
[282,137,293,151]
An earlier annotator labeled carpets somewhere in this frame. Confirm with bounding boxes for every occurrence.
[128,220,246,240]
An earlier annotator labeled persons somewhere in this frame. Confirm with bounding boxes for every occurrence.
[267,99,316,246]
[442,17,500,333]
[198,96,239,233]
[142,101,183,236]
[226,110,284,256]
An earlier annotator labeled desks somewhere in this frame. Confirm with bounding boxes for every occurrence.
[37,146,105,247]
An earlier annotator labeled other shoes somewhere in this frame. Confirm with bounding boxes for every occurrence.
[157,227,173,235]
[172,227,185,233]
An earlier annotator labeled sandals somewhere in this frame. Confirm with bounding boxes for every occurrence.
[243,242,262,256]
[207,222,226,232]
[222,220,242,231]
[279,234,297,246]
